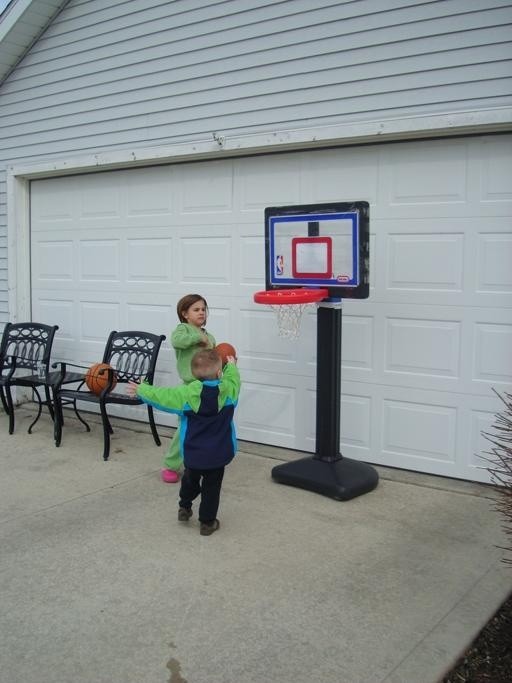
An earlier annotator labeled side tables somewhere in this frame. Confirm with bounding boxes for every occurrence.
[12,369,92,436]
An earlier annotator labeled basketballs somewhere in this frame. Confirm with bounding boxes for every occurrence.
[85,363,117,394]
[216,343,235,364]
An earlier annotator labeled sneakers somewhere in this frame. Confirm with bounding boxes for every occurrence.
[162,466,180,484]
[200,517,220,536]
[177,506,193,521]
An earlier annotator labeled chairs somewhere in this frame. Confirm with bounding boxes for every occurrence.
[1,320,58,433]
[55,329,166,460]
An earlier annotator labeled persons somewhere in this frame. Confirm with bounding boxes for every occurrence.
[125,349,241,535]
[162,295,216,482]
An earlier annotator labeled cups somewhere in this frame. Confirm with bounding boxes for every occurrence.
[37,364,46,379]
